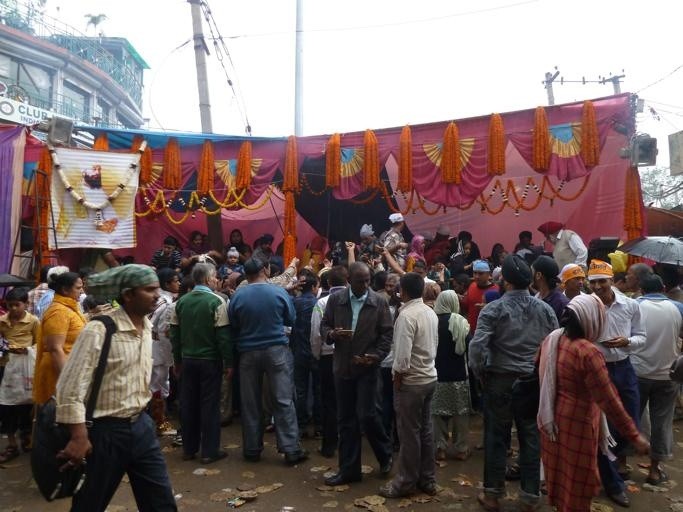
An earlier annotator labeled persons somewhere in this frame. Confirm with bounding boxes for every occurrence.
[405,235,428,276]
[212,274,233,427]
[253,234,274,263]
[587,259,640,508]
[484,245,506,270]
[81,293,103,314]
[384,273,400,293]
[452,274,471,298]
[536,293,651,511]
[76,266,91,313]
[613,273,628,294]
[460,260,499,339]
[558,263,585,298]
[379,272,438,497]
[493,250,508,268]
[149,236,181,273]
[468,254,560,512]
[169,263,234,464]
[319,261,394,486]
[181,230,224,268]
[172,277,195,303]
[318,271,330,297]
[382,247,436,284]
[447,231,480,262]
[537,221,588,281]
[519,231,542,254]
[317,252,336,279]
[36,266,70,321]
[449,238,473,287]
[371,271,390,306]
[459,231,481,264]
[236,257,300,293]
[622,262,683,448]
[145,267,179,435]
[55,160,121,246]
[504,254,572,480]
[428,256,450,291]
[30,272,88,452]
[310,241,356,458]
[429,289,473,460]
[0,287,40,462]
[357,243,408,274]
[480,291,499,311]
[422,284,441,307]
[223,229,251,263]
[380,287,403,452]
[55,264,178,511]
[384,213,407,273]
[228,256,309,464]
[292,272,324,440]
[217,247,246,279]
[25,265,50,314]
[224,268,243,288]
[357,223,386,268]
[425,224,450,261]
[665,269,683,301]
[630,274,683,484]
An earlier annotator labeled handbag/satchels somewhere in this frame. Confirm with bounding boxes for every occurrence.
[30,315,116,503]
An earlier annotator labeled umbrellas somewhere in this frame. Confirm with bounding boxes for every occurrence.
[618,235,682,266]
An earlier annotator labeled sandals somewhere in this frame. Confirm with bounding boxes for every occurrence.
[646,468,668,486]
[457,449,473,460]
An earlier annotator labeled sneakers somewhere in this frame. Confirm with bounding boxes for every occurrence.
[161,429,178,436]
[379,482,416,497]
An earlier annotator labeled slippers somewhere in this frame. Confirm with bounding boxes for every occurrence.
[478,492,499,511]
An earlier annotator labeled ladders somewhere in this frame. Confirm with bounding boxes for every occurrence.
[1,168,63,302]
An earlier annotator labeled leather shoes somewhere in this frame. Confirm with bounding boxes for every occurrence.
[381,458,392,473]
[288,452,309,462]
[23,441,31,452]
[200,453,226,464]
[325,472,360,485]
[0,445,19,464]
[612,493,631,510]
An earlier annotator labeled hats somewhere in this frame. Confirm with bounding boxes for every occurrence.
[227,246,239,258]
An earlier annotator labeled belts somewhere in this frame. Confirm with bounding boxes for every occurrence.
[602,357,630,371]
